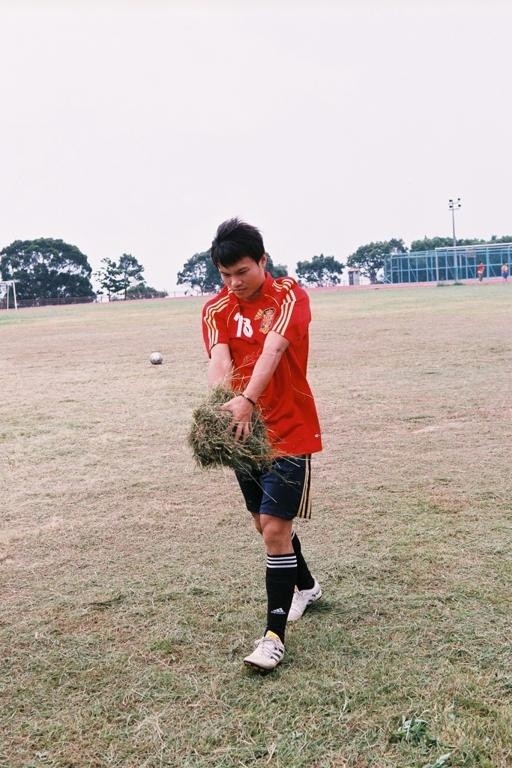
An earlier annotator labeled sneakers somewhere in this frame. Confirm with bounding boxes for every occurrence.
[287,576,322,622]
[243,630,285,670]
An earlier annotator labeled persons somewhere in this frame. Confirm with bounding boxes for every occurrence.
[501,263,509,281]
[474,260,486,283]
[200,221,324,675]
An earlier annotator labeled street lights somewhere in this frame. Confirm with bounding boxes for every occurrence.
[448,196,462,283]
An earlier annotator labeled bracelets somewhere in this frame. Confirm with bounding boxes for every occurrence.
[237,390,259,408]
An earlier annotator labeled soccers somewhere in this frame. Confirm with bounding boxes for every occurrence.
[149,350,164,364]
